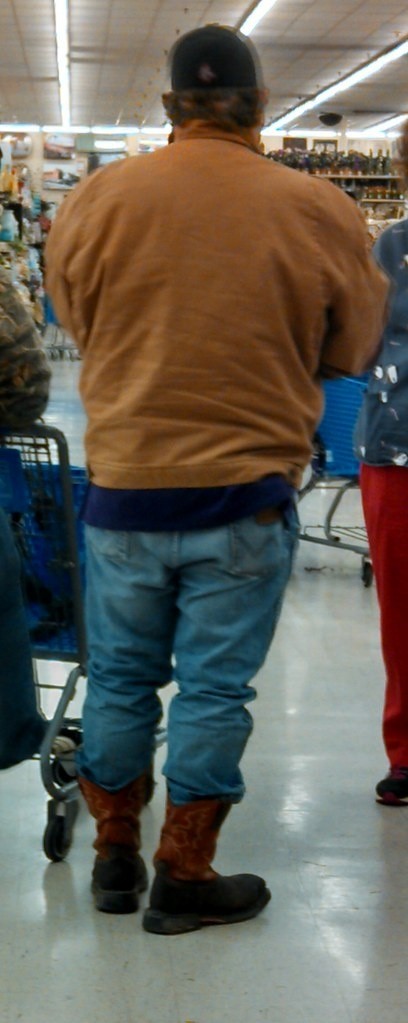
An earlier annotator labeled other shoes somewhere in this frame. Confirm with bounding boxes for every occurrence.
[91,853,147,915]
[143,871,271,936]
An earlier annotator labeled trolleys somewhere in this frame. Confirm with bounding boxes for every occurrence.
[2,423,168,862]
[295,376,375,589]
[41,291,78,363]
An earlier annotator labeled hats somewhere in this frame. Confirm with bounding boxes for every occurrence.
[169,23,257,88]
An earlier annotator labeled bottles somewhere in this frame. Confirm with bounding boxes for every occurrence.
[269,146,406,220]
[1,210,16,243]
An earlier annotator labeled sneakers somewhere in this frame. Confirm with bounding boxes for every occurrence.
[376,766,408,805]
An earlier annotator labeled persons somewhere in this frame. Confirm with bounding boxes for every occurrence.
[42,23,391,936]
[353,119,408,804]
[0,263,51,771]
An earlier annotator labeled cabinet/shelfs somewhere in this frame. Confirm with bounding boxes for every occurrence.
[264,146,408,235]
[0,132,81,314]
